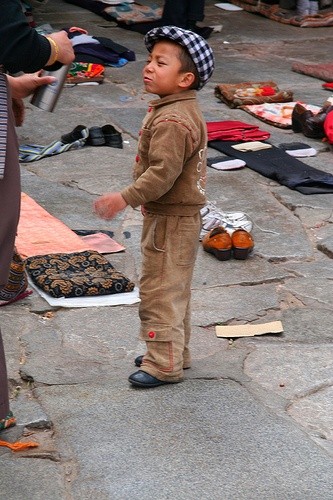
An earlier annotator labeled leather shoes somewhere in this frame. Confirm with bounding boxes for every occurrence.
[292,103,313,133]
[102,124,123,149]
[86,127,105,145]
[128,370,170,387]
[304,106,332,139]
[135,355,145,366]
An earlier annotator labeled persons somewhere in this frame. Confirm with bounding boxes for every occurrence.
[93,27,215,390]
[0,0,75,431]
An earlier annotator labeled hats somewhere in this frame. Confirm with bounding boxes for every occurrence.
[144,26,215,91]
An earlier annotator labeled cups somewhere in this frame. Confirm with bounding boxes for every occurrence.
[30,61,71,113]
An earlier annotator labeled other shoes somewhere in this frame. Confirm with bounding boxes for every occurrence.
[61,125,85,144]
[202,226,232,261]
[232,229,254,260]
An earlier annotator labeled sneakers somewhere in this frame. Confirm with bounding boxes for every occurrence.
[200,203,248,229]
[198,216,253,242]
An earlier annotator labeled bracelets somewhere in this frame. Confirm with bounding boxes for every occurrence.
[44,35,60,66]
